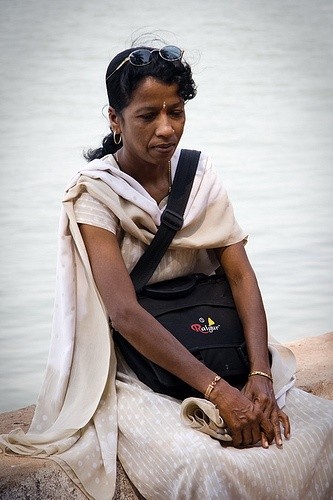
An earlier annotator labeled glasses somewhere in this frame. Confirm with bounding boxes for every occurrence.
[105,45,183,79]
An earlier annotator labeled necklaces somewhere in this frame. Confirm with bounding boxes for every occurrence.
[113,149,172,194]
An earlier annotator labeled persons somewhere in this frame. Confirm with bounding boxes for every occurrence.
[0,44,333,499]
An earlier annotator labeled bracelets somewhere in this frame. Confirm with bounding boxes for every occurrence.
[247,370,274,382]
[204,374,222,400]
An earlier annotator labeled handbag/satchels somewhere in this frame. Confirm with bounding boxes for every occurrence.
[109,272,272,402]
